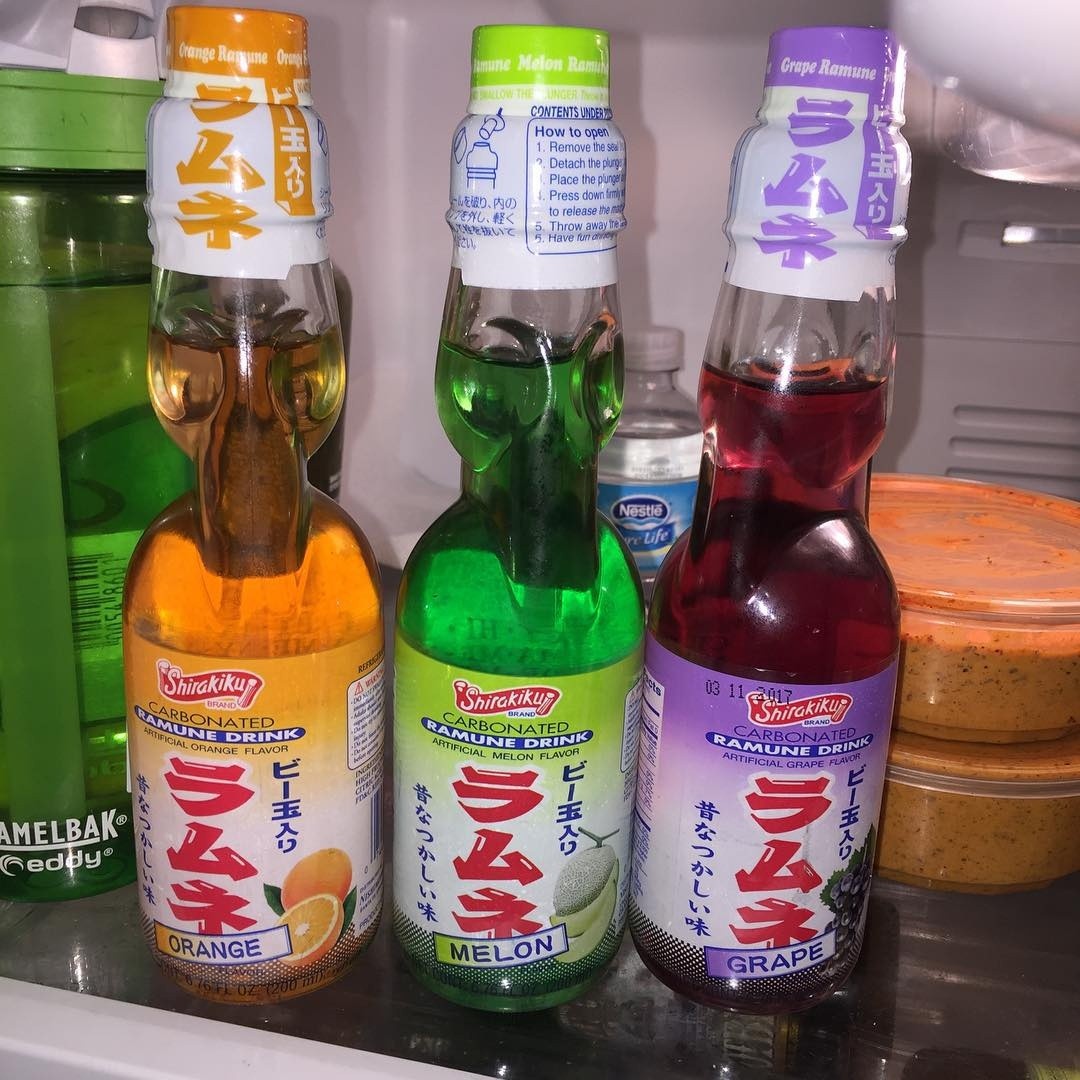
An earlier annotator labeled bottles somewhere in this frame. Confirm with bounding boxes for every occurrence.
[114,6,384,1000]
[390,24,643,1015]
[589,324,710,605]
[0,65,195,902]
[623,29,904,1011]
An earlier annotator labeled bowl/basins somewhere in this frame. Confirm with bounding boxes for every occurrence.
[821,468,1080,895]
